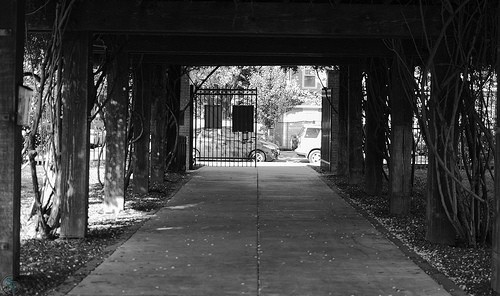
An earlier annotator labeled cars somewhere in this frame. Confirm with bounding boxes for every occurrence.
[193,127,280,162]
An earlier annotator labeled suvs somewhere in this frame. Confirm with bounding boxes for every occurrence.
[292,123,321,163]
